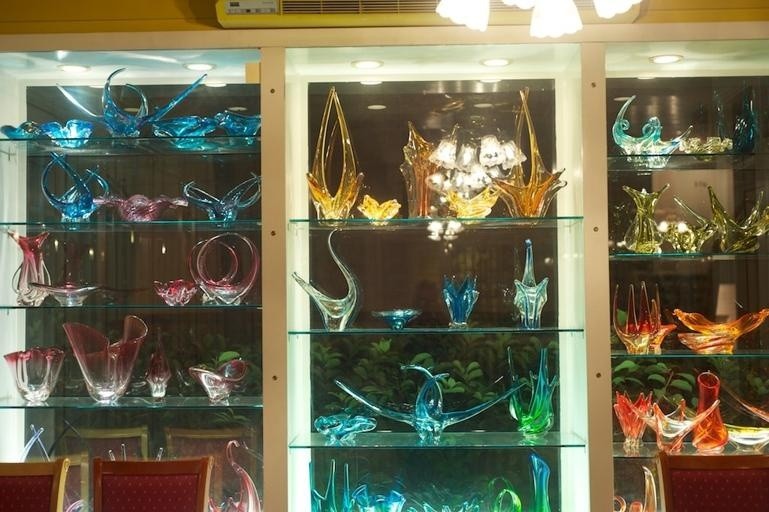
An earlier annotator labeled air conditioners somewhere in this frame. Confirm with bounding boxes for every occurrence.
[214,0,645,31]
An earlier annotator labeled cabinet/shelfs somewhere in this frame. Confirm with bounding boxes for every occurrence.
[555,15,768,512]
[1,29,311,512]
[257,25,612,512]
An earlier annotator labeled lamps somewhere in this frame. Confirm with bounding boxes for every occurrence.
[435,0,645,36]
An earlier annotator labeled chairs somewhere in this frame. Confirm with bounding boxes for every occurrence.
[655,451,768,512]
[1,456,70,512]
[92,453,216,512]
